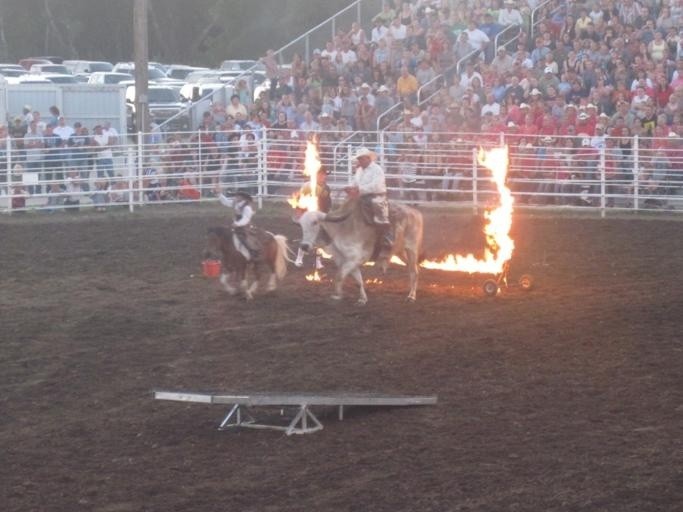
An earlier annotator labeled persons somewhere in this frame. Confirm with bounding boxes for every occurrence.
[217,185,260,260]
[344,146,396,251]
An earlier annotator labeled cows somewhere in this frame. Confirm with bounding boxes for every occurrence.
[288,196,424,308]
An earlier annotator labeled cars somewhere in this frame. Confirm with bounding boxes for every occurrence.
[0,56,296,144]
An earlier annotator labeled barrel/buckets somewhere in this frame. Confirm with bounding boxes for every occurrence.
[202,260,221,276]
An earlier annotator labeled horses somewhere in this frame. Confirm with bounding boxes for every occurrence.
[206,224,298,296]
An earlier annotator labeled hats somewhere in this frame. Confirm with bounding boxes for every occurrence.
[516,32,527,46]
[227,187,253,203]
[540,137,556,143]
[504,0,517,8]
[351,147,379,163]
[594,123,605,131]
[318,112,333,121]
[497,46,506,52]
[564,103,598,114]
[519,102,529,109]
[448,103,462,110]
[377,85,390,92]
[400,108,416,118]
[46,115,106,130]
[12,104,32,123]
[359,83,371,88]
[313,49,321,55]
[596,113,610,121]
[529,88,542,96]
[581,139,591,147]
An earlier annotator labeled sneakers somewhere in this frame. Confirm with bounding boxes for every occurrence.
[384,228,395,245]
